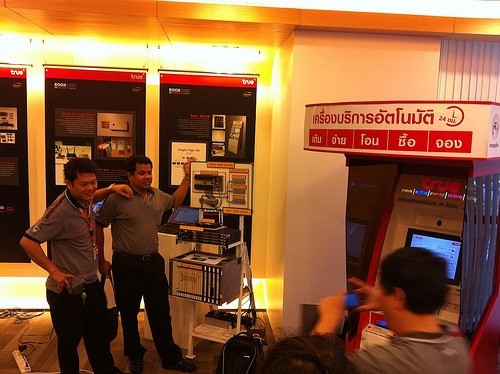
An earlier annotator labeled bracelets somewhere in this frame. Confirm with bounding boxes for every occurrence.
[184,173,189,181]
[108,184,115,192]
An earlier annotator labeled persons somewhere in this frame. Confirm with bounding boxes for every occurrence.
[96,156,197,374]
[312,246,468,374]
[251,327,363,374]
[20,157,133,374]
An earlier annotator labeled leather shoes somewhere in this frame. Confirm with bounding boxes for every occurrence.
[161,358,196,372]
[128,359,143,374]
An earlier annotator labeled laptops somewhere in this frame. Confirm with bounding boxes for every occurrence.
[158,205,199,234]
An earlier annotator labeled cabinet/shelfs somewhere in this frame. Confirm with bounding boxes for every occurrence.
[167,209,259,360]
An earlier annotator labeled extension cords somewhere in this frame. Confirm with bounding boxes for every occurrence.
[12,349,31,373]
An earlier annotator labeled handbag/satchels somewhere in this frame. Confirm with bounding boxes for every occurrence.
[100,273,119,342]
[214,332,260,374]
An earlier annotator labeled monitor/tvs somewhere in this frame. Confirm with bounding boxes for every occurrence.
[404,227,463,287]
